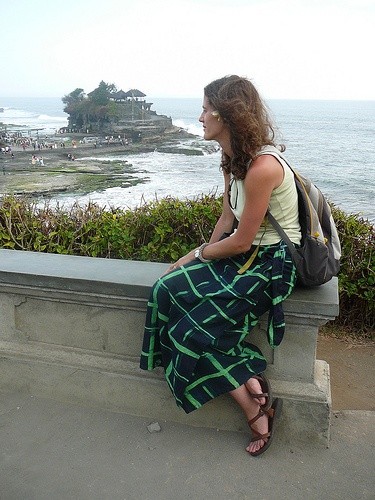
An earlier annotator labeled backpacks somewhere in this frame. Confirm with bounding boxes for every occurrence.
[245,150,343,287]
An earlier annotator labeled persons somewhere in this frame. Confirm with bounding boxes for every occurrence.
[139,74,303,457]
[0,126,142,168]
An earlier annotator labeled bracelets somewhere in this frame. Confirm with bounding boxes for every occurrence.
[199,242,213,263]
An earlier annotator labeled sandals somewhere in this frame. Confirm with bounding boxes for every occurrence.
[245,371,274,412]
[247,398,284,456]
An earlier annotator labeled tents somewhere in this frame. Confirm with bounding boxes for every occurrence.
[108,89,145,103]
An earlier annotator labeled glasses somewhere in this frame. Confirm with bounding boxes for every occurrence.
[227,177,239,210]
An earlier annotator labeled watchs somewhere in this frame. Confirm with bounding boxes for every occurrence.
[194,249,207,261]
